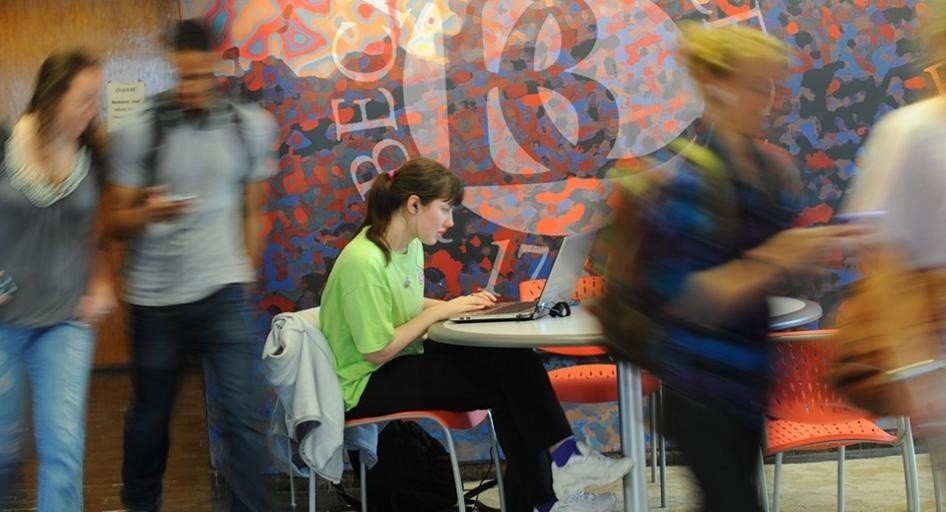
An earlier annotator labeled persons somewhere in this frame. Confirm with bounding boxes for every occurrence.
[838,2,944,461]
[96,18,280,512]
[635,20,881,511]
[0,46,120,511]
[316,157,637,512]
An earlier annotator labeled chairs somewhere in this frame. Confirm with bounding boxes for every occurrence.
[274,271,923,512]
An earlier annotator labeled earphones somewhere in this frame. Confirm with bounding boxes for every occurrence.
[413,203,419,211]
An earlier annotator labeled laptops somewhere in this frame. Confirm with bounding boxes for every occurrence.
[448,230,592,325]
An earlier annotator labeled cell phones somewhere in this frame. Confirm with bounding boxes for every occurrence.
[830,211,890,231]
[164,191,195,202]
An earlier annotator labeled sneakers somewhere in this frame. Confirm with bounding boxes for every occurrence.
[531,439,636,512]
